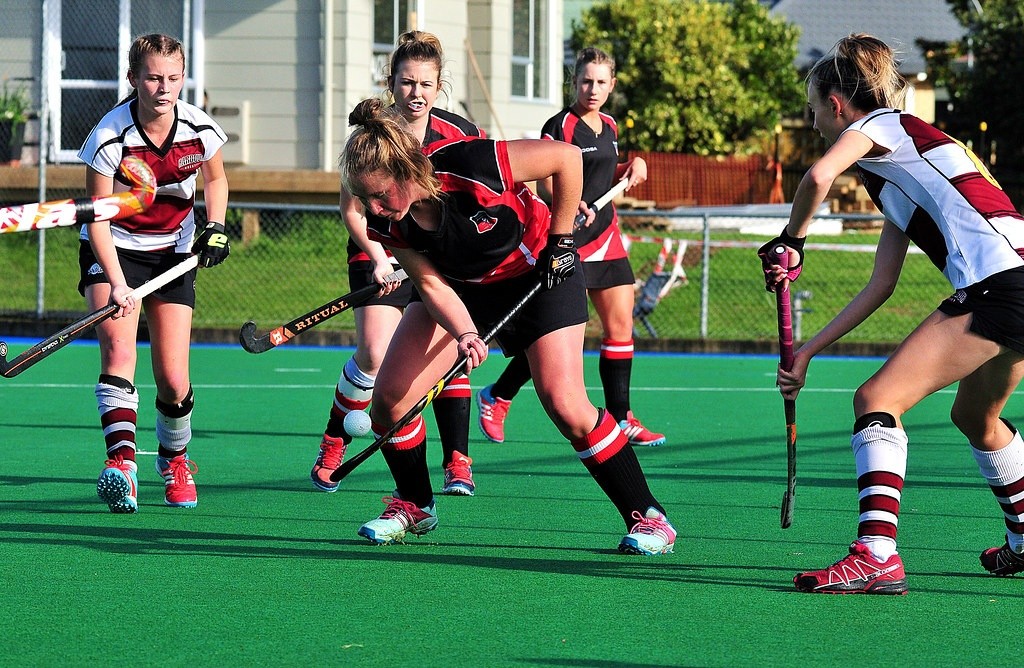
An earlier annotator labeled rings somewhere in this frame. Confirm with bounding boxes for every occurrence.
[635,181,639,184]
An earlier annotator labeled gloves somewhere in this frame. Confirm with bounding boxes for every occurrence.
[538,234,574,287]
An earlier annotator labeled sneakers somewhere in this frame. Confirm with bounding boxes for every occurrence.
[155,452,197,508]
[358,488,439,546]
[476,383,512,444]
[97,454,138,514]
[793,540,908,595]
[618,506,677,555]
[311,434,348,492]
[443,450,475,496]
[619,410,666,446]
[979,533,1024,576]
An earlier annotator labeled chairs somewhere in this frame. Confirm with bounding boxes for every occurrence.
[632,271,672,339]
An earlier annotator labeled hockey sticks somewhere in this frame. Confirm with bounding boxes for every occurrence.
[238,268,409,354]
[0,252,201,378]
[573,177,630,235]
[771,244,796,529]
[329,281,546,483]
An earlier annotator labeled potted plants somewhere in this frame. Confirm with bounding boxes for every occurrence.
[0,70,36,164]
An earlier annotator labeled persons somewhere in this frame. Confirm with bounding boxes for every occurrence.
[311,31,491,496]
[77,33,230,513]
[476,46,666,445]
[338,99,678,556]
[756,33,1024,595]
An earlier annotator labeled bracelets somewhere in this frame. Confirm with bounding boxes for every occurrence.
[457,332,480,340]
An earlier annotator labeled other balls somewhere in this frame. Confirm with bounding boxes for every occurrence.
[343,409,372,437]
